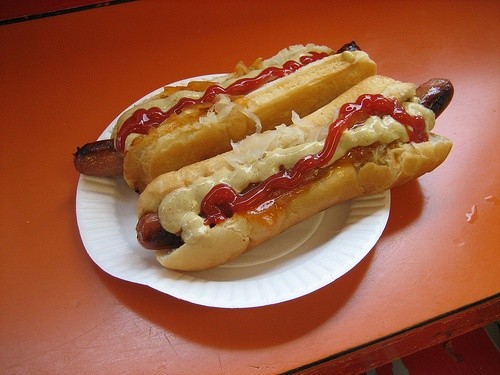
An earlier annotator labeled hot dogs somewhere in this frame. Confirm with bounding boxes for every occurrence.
[72,41,454,272]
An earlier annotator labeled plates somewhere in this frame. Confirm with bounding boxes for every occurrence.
[76,71,391,309]
